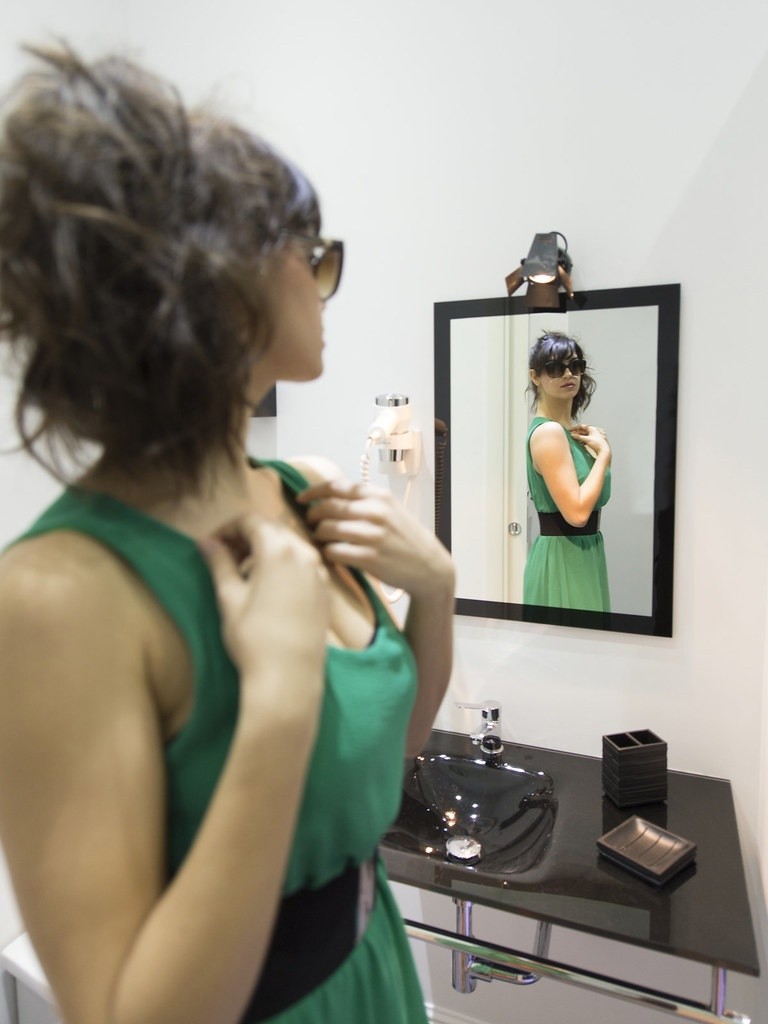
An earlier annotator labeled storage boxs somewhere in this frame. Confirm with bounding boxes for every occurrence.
[602,729,670,808]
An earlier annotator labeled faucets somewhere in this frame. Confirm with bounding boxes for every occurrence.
[453,699,503,749]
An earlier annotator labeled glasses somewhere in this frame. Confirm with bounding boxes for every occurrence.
[542,360,587,379]
[280,226,345,301]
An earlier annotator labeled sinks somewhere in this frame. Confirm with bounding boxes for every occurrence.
[376,750,563,879]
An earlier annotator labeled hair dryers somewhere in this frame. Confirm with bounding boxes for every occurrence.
[367,391,411,481]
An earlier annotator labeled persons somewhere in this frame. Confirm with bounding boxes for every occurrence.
[523,331,612,612]
[0,41,455,1024]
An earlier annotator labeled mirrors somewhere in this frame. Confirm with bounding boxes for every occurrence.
[435,283,681,637]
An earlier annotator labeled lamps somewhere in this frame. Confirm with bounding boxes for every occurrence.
[504,231,574,311]
